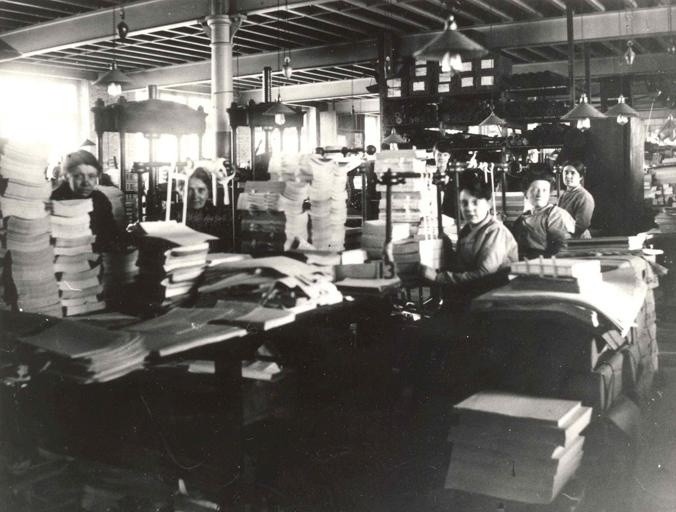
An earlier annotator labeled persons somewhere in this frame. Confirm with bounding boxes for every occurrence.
[428,141,458,219]
[508,172,576,264]
[414,178,522,316]
[557,158,597,242]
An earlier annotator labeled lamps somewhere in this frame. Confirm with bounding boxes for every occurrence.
[280,2,293,79]
[480,48,507,130]
[624,1,635,66]
[262,2,295,124]
[93,2,134,96]
[91,1,675,153]
[604,1,639,123]
[381,2,407,149]
[117,2,130,40]
[665,2,675,54]
[558,2,608,131]
[412,2,488,76]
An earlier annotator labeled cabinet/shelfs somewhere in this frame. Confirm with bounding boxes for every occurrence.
[568,116,645,239]
[377,79,592,225]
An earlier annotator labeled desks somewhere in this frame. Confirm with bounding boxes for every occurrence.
[1,270,431,509]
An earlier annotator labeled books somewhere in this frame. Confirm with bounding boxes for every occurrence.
[475,233,664,336]
[353,139,446,305]
[439,388,594,506]
